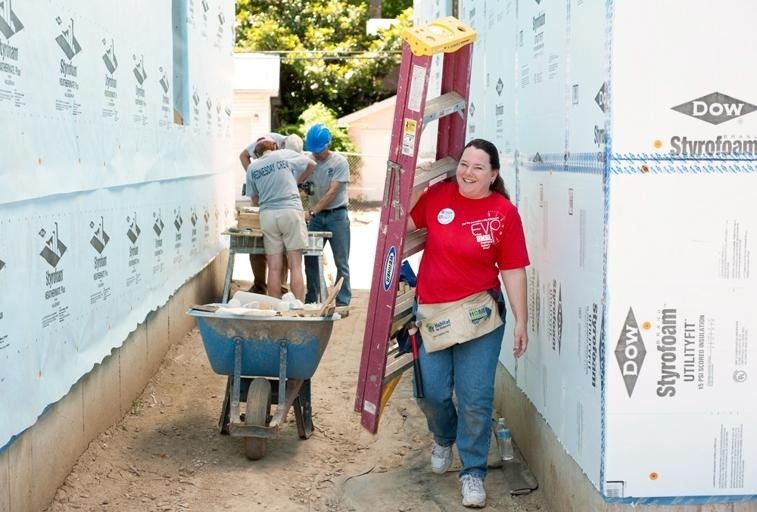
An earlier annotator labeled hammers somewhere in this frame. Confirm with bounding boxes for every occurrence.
[402,320,424,398]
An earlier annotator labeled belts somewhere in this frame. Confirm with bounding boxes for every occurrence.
[320,206,346,213]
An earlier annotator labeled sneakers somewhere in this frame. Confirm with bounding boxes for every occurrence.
[431,443,453,474]
[461,474,486,508]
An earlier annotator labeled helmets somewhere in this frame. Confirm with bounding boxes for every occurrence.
[253,124,332,153]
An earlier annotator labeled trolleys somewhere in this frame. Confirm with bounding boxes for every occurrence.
[185,302,342,461]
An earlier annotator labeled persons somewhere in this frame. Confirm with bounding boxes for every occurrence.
[300,123,353,314]
[246,135,317,303]
[237,132,304,293]
[404,138,531,508]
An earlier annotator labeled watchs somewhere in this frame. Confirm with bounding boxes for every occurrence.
[308,207,316,218]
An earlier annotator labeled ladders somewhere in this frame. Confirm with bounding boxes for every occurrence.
[354,15,477,435]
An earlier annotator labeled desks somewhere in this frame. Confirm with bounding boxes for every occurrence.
[221,227,333,304]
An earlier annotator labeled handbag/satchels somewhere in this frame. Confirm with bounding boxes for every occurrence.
[415,290,504,354]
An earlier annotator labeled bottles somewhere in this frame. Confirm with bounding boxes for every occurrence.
[494,417,513,460]
